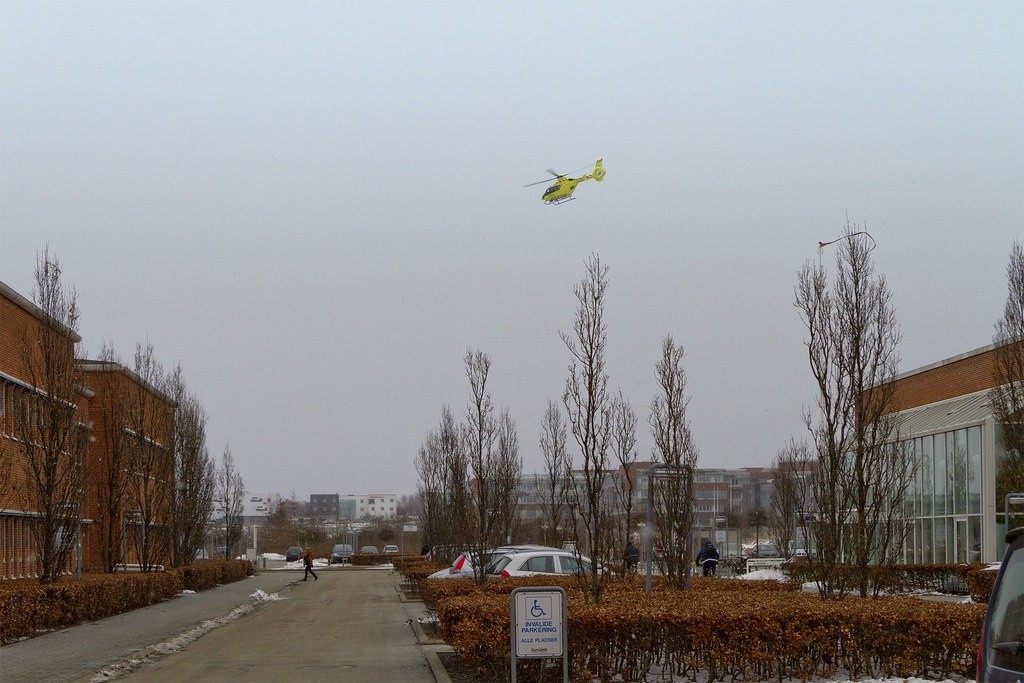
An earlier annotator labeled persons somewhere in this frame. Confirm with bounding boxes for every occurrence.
[303,547,318,582]
[622,542,638,572]
[421,546,429,556]
[696,540,719,577]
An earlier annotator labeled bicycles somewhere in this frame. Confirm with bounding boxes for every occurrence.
[698,556,719,578]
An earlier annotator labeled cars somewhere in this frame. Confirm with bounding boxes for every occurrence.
[381,545,401,555]
[329,543,356,563]
[285,547,304,561]
[427,546,570,578]
[483,551,615,575]
[358,545,378,558]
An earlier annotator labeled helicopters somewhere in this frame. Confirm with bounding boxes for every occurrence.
[523,153,609,210]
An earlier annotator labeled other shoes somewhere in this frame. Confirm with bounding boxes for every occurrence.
[303,578,307,581]
[314,577,319,581]
